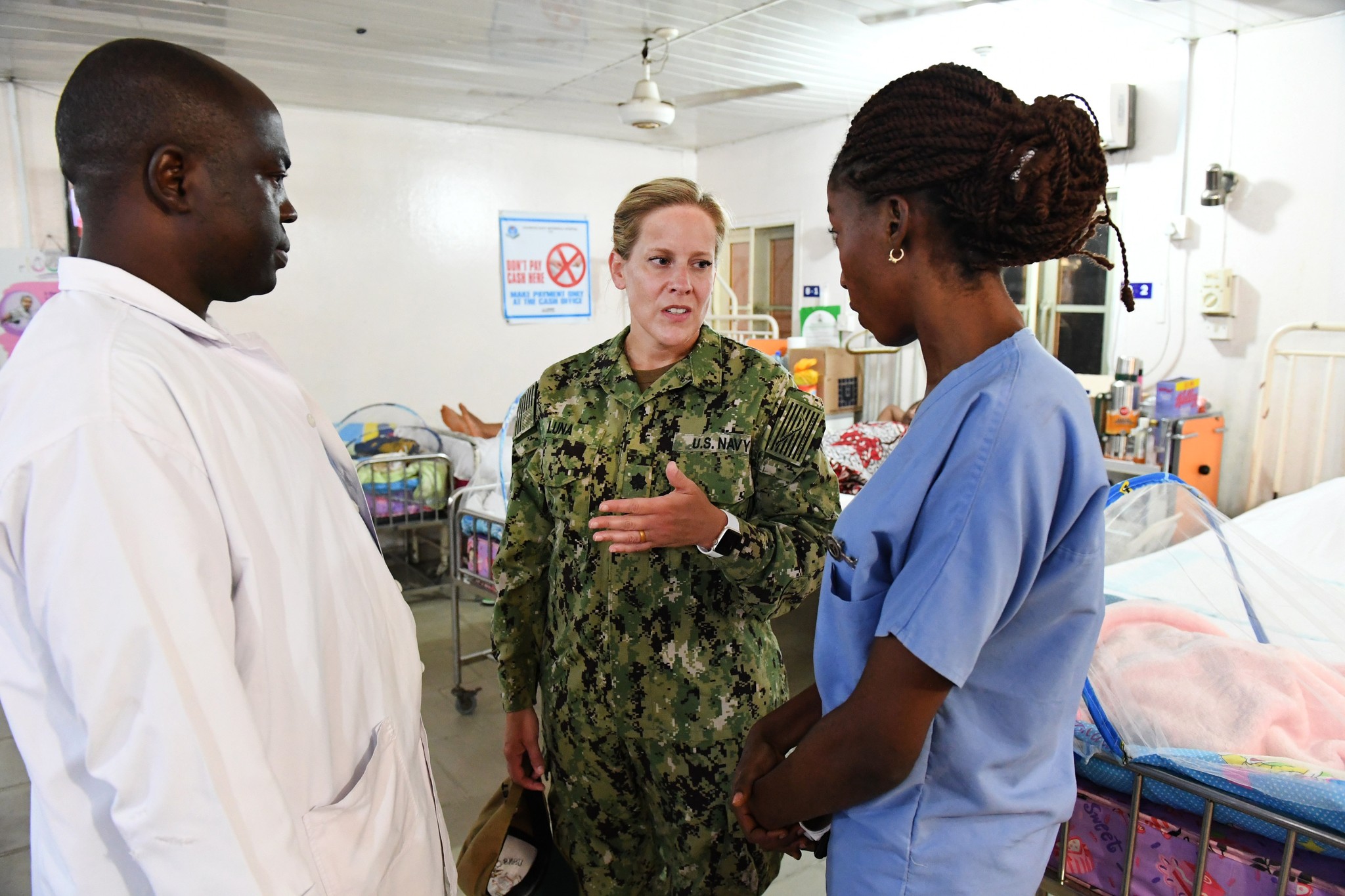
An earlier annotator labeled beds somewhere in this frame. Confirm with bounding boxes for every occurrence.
[333,313,1345,896]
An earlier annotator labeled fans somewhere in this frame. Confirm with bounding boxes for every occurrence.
[466,37,808,129]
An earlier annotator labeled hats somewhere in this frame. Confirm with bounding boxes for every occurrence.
[454,763,583,896]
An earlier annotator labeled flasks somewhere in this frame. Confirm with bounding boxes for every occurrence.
[1093,356,1140,460]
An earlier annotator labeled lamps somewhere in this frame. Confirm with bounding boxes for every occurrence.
[1200,164,1238,207]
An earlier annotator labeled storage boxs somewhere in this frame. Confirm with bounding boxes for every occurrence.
[791,346,865,415]
[1156,379,1201,465]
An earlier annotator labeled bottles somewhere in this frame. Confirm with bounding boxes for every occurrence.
[1131,416,1158,466]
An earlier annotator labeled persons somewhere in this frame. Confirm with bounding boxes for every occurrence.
[821,398,923,496]
[440,403,503,439]
[490,177,843,896]
[1,36,467,895]
[729,61,1136,896]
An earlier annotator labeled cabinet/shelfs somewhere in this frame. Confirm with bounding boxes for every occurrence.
[1102,412,1225,542]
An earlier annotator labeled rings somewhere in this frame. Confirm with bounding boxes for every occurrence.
[638,530,646,543]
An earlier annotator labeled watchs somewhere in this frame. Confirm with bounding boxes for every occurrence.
[697,509,743,559]
